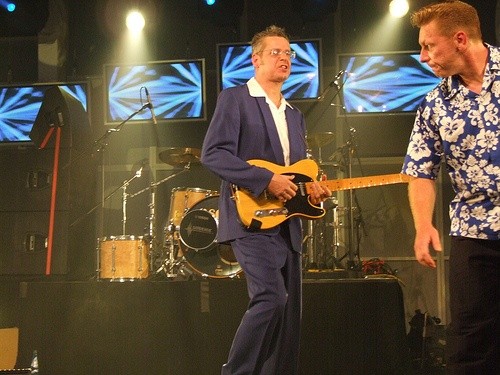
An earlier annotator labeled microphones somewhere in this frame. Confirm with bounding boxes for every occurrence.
[145,87,160,129]
[315,70,344,104]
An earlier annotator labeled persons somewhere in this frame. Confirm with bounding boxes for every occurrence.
[201,26,332,375]
[400,0,500,375]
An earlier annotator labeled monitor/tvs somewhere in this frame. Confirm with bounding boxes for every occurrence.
[0,80,92,146]
[102,58,209,126]
[335,49,451,117]
[215,37,324,104]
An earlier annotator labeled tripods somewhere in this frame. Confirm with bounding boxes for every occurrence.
[77,84,369,280]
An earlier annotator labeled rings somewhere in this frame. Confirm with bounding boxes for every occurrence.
[281,192,287,195]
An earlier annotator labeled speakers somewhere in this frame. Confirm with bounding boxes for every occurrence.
[30,86,91,163]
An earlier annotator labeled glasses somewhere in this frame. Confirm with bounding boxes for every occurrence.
[256,48,296,59]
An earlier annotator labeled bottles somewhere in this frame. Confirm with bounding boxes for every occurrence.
[30,350,39,375]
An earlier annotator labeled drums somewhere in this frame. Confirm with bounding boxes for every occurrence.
[97,235,149,280]
[180,194,245,279]
[166,187,220,248]
[180,209,218,253]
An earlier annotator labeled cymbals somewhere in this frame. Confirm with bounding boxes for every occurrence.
[159,148,201,168]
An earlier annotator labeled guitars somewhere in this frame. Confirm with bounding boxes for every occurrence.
[233,158,408,229]
